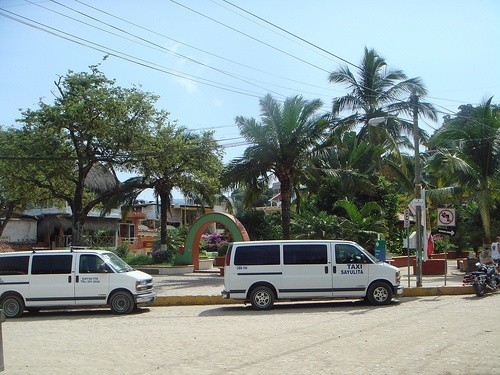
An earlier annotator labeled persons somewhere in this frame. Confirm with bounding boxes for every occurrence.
[471,235,500,273]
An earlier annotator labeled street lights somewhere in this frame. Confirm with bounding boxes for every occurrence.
[368,96,423,287]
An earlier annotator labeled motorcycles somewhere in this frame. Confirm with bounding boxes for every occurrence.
[462,262,500,298]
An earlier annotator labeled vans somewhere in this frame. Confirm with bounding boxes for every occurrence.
[221,240,403,310]
[0,247,154,319]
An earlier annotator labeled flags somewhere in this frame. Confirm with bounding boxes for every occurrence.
[423,189,434,262]
